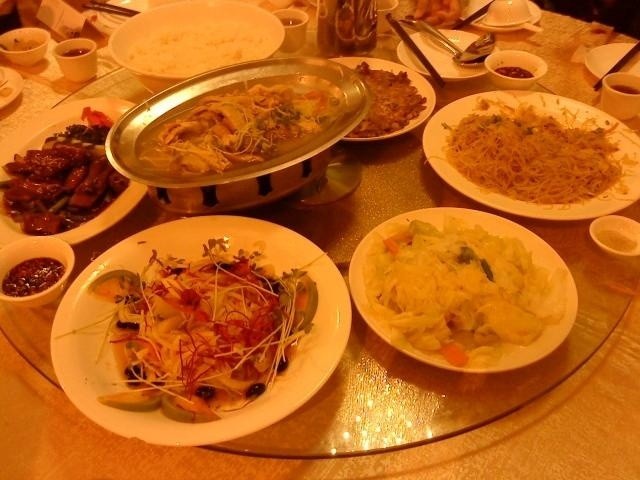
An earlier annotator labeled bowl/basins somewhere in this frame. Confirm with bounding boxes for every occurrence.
[483,49,550,95]
[2,236,75,308]
[1,27,51,67]
[108,1,285,95]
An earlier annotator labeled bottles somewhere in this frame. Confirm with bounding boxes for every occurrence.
[320,0,378,57]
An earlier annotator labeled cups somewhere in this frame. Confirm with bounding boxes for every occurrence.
[269,8,309,54]
[481,0,533,25]
[54,39,98,81]
[602,73,638,126]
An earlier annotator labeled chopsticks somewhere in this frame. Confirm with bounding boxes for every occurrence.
[452,0,495,30]
[593,43,638,93]
[383,12,445,90]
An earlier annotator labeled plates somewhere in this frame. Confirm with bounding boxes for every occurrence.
[421,90,639,222]
[104,45,370,220]
[396,26,500,83]
[323,52,436,140]
[583,42,638,83]
[347,208,579,374]
[1,96,158,244]
[47,216,361,448]
[461,2,543,31]
[1,68,24,110]
